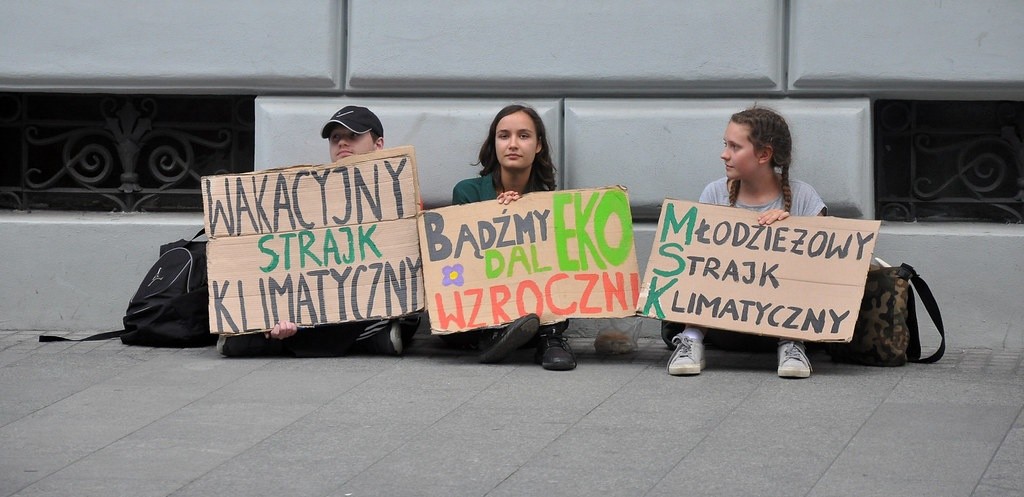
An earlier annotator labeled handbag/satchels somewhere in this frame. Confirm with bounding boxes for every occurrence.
[829,263,947,368]
[593,317,643,358]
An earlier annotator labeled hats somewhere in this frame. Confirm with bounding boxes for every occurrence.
[321,106,384,139]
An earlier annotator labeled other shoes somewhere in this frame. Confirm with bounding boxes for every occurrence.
[217,334,244,357]
[374,321,403,357]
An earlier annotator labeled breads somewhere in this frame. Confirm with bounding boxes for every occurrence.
[594,333,636,354]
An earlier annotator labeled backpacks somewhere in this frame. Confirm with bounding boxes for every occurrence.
[116,227,219,349]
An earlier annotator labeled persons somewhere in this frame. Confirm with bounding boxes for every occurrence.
[671,107,827,378]
[218,105,422,356]
[453,103,576,372]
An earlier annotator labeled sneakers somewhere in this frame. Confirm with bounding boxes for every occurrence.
[776,340,814,378]
[476,313,541,365]
[541,333,578,371]
[665,332,707,376]
